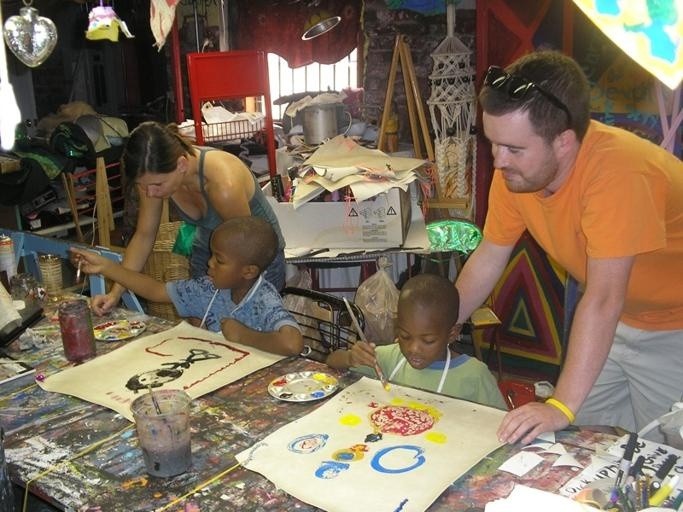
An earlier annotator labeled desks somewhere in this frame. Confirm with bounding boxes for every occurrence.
[277,197,435,354]
[0,276,682,512]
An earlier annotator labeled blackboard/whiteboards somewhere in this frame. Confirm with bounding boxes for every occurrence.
[363,1,683,157]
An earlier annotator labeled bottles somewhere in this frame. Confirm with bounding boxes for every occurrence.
[57,299,95,359]
[0,234,15,281]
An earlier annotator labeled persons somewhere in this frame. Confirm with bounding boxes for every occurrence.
[320,273,509,408]
[71,214,305,356]
[89,122,287,335]
[449,48,683,453]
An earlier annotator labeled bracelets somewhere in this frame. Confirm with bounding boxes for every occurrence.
[544,398,575,425]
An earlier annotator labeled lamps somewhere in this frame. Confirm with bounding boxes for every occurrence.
[271,0,342,43]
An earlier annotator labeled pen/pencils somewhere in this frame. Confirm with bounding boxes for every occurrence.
[611,470,682,512]
[507,395,515,409]
[75,261,82,284]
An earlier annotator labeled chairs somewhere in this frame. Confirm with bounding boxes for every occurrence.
[494,376,542,419]
[403,216,506,392]
[271,286,367,374]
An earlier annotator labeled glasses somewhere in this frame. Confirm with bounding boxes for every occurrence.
[482,63,572,126]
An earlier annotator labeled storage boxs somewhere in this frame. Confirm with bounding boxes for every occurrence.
[252,157,416,255]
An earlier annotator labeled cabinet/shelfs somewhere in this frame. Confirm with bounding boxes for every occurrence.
[0,129,137,310]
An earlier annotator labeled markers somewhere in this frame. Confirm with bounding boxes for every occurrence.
[613,433,677,487]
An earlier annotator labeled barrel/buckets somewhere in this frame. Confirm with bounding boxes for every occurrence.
[301,102,353,147]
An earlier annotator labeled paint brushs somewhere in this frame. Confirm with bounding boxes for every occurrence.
[341,295,392,392]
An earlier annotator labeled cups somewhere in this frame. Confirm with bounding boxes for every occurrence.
[9,272,38,320]
[38,254,64,296]
